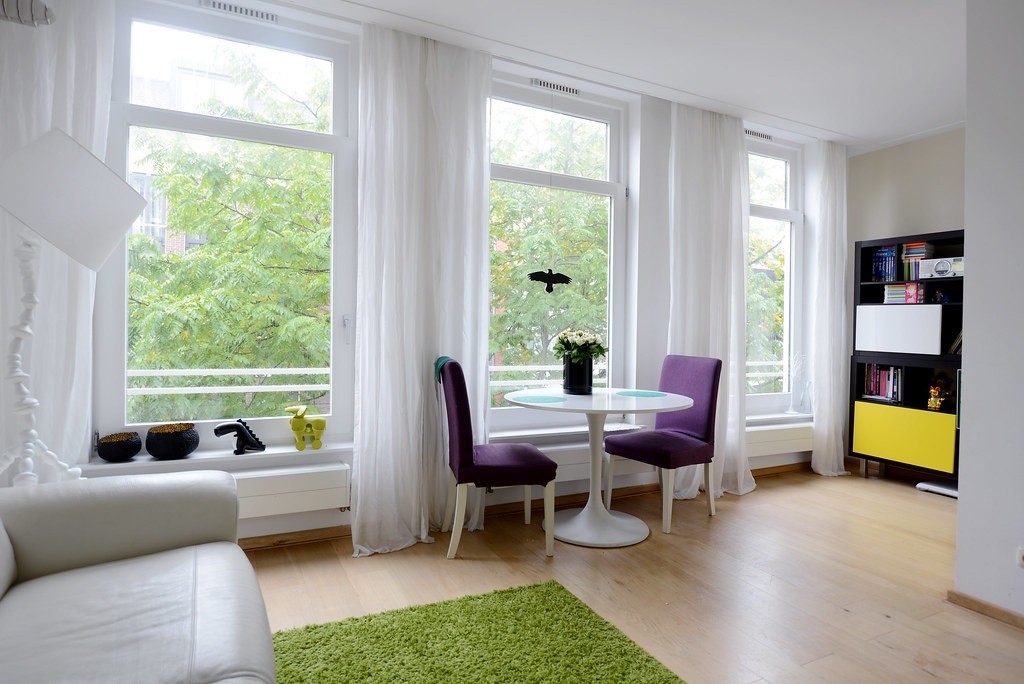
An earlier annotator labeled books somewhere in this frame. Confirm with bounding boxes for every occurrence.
[902,243,937,282]
[870,245,896,281]
[864,361,904,401]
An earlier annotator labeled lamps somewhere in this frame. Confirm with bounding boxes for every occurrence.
[1,127,149,488]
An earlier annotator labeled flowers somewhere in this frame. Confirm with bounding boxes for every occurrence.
[552,330,610,364]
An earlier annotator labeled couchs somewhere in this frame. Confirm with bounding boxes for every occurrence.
[0,469,277,684]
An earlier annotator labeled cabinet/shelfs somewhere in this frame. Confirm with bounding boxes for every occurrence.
[847,229,965,484]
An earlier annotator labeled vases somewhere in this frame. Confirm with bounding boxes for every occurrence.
[146,423,201,460]
[96,431,142,462]
[562,354,593,396]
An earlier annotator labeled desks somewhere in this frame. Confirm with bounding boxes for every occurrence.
[503,387,694,548]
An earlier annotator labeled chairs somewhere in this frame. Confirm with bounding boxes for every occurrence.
[603,354,724,534]
[435,355,559,559]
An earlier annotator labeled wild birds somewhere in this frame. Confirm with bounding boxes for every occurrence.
[527,269,572,294]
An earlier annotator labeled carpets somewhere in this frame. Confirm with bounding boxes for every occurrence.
[270,579,688,684]
